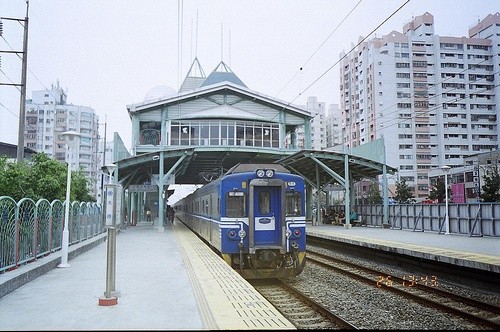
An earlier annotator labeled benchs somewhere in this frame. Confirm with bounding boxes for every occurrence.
[332,213,363,226]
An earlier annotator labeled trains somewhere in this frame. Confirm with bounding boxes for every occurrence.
[173,163,307,281]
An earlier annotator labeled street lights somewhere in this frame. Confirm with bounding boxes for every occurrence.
[106,164,117,183]
[441,166,452,235]
[57,129,82,268]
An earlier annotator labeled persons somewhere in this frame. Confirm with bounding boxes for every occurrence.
[145,209,152,223]
[312,206,359,226]
[167,204,175,224]
[123,208,127,223]
